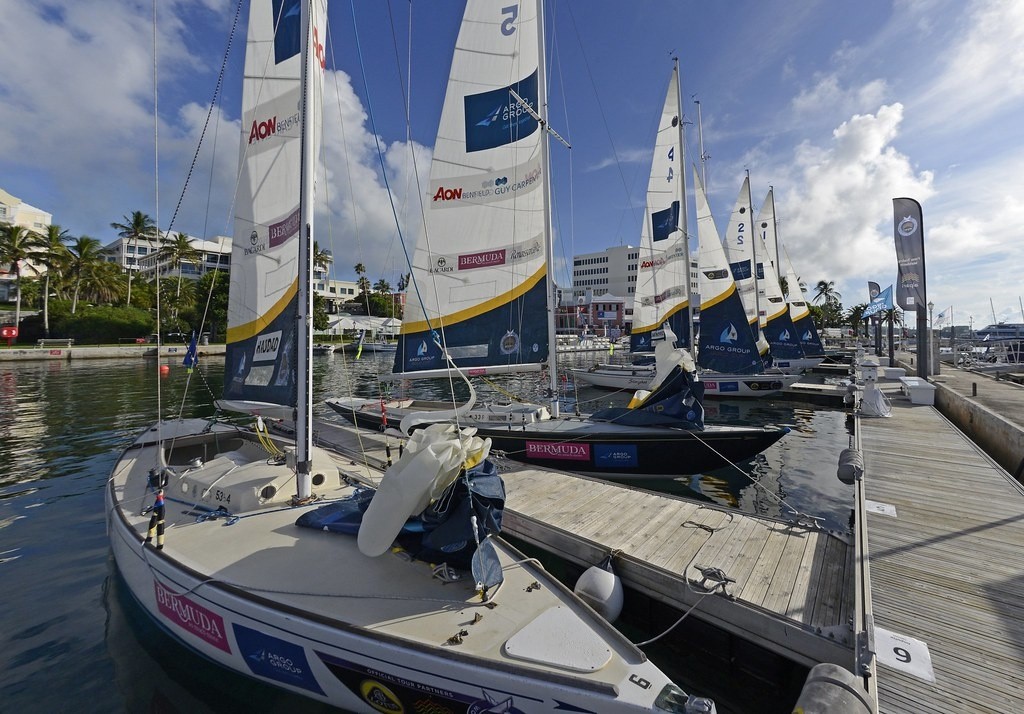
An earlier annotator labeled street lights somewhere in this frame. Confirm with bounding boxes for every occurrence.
[927,300,935,376]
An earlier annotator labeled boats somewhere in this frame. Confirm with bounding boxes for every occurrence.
[312,342,389,357]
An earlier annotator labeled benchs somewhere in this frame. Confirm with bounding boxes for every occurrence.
[868,347,875,355]
[897,376,938,406]
[883,367,908,380]
[32,337,75,349]
[878,356,891,366]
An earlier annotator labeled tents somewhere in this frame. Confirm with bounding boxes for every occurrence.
[323,317,400,340]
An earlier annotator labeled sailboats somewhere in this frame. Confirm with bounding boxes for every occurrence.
[324,0,792,482]
[942,297,1024,386]
[563,50,844,398]
[104,2,720,714]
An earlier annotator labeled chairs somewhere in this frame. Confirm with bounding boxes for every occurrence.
[378,326,386,341]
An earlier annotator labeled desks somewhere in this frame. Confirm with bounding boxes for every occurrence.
[378,332,395,341]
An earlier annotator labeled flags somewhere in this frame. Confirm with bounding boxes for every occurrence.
[859,285,893,317]
[182,330,199,370]
[934,307,952,327]
